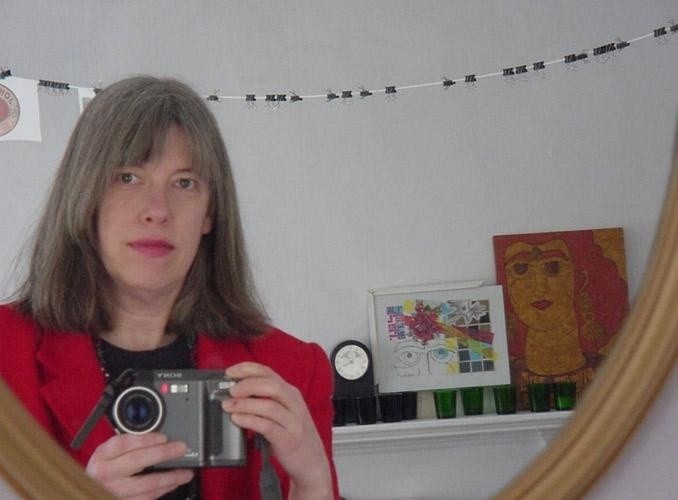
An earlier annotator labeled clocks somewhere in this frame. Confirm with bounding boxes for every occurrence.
[329,341,377,427]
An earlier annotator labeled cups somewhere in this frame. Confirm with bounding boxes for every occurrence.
[331,390,418,427]
[431,380,575,419]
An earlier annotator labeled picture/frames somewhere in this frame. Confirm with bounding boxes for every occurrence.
[367,280,511,394]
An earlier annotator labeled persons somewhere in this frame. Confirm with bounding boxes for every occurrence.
[1,74,339,500]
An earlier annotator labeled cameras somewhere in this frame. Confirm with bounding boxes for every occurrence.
[113,369,246,470]
